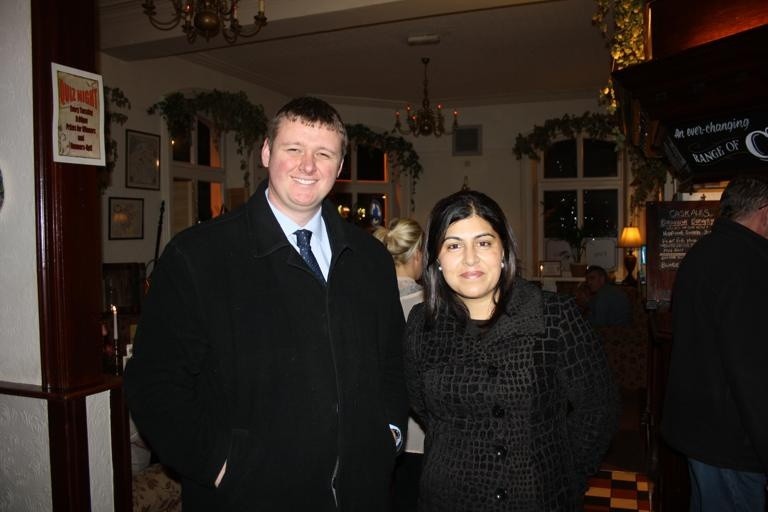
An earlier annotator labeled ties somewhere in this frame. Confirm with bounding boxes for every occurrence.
[294,229,327,288]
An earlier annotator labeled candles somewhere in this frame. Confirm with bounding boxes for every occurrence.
[109,303,121,340]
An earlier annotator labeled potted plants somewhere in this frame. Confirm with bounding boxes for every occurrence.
[557,221,590,275]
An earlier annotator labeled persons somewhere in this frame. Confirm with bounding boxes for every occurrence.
[122,97,407,511]
[585,266,631,326]
[655,179,767,512]
[373,216,425,455]
[404,190,617,512]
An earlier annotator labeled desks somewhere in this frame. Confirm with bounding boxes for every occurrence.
[525,276,587,291]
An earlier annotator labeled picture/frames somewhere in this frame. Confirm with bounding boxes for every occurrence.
[538,259,563,277]
[123,127,162,192]
[107,194,145,241]
[450,123,485,158]
[48,61,108,169]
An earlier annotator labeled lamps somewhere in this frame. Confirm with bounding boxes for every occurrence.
[617,224,644,281]
[138,0,270,45]
[392,48,461,139]
